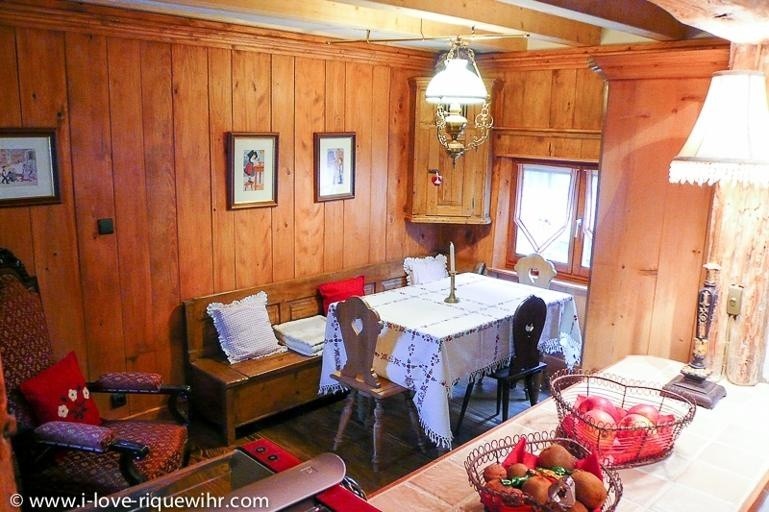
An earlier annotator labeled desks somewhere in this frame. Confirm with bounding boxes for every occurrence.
[328,271,574,454]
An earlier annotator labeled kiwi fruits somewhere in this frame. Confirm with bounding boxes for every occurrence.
[483,445,607,511]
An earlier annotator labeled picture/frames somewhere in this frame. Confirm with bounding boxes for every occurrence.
[225,130,357,211]
[0,126,65,209]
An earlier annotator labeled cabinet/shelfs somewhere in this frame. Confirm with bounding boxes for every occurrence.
[408,76,504,225]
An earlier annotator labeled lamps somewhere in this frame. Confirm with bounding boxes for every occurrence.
[423,33,496,170]
[657,68,769,411]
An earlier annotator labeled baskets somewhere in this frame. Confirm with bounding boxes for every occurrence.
[464,430,623,512]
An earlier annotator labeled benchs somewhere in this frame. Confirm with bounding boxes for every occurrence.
[181,252,444,448]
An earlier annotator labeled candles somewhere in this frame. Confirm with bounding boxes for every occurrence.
[449,241,456,271]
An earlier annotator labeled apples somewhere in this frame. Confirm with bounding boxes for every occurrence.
[578,396,660,454]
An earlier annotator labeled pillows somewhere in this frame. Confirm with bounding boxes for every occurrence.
[206,290,288,367]
[0,247,194,512]
[317,275,367,316]
[17,350,103,429]
